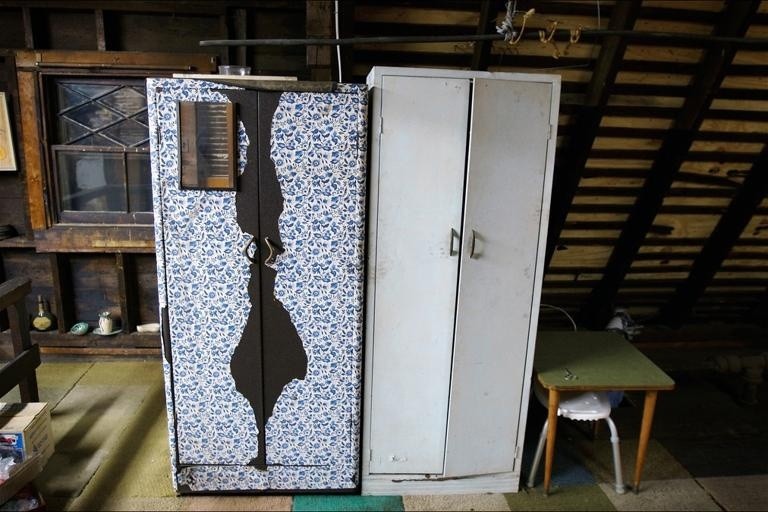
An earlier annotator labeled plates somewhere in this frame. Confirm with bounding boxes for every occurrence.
[93,327,122,336]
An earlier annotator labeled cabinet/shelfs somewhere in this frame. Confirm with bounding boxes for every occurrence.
[141,74,372,498]
[362,64,566,499]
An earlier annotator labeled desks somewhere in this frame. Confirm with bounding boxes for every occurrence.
[534,326,679,497]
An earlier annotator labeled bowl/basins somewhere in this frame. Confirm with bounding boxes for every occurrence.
[217,65,253,76]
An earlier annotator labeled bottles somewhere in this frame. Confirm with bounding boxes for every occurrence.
[97,312,114,333]
[29,294,55,332]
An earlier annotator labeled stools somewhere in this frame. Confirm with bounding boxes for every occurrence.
[523,370,634,497]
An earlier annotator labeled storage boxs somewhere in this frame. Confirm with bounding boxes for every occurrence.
[0,396,60,471]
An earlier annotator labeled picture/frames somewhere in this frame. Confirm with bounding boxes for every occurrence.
[1,88,22,173]
